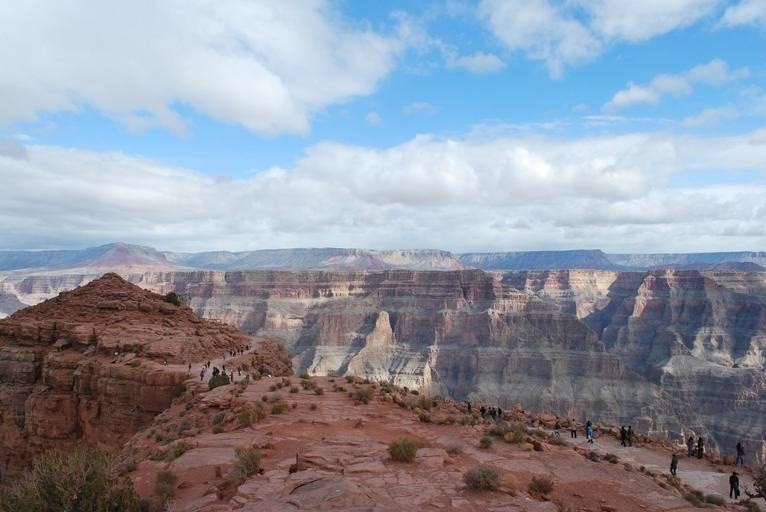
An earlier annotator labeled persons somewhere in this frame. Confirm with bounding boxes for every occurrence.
[669,454,678,476]
[729,471,740,499]
[627,426,635,446]
[620,425,627,447]
[554,421,562,437]
[570,418,579,439]
[697,437,704,459]
[162,344,274,382]
[586,420,593,443]
[467,400,503,419]
[687,436,694,456]
[734,442,745,465]
